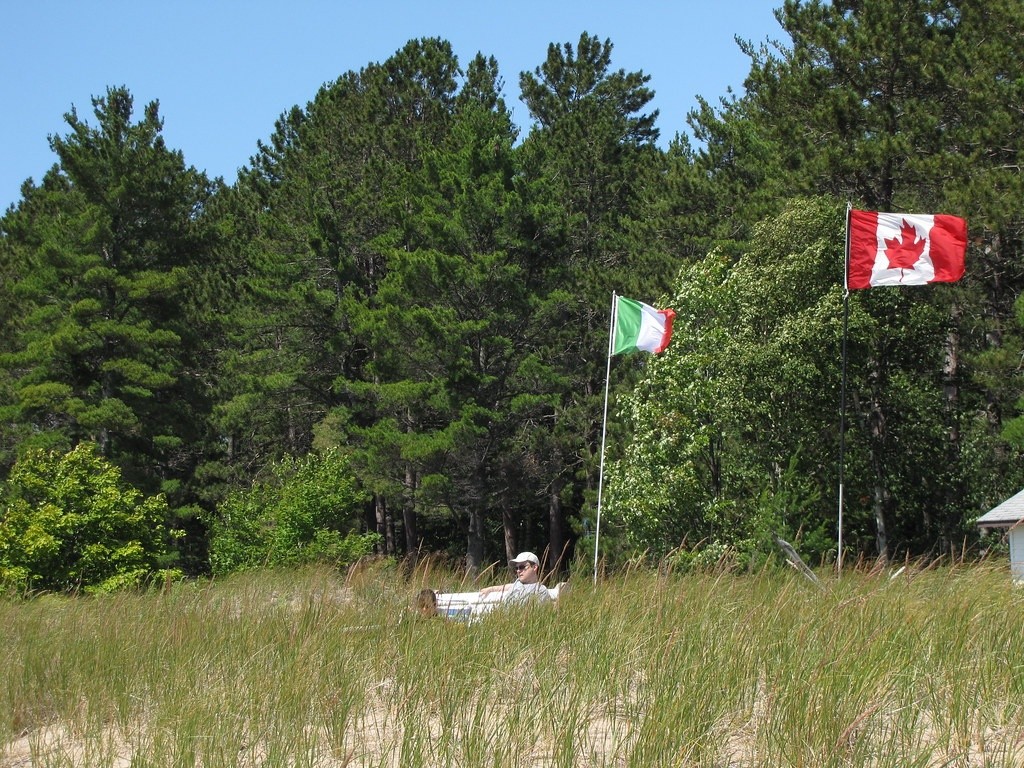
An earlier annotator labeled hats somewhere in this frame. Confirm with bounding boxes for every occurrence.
[509,552,539,565]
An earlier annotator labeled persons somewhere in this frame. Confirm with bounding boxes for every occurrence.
[479,551,551,602]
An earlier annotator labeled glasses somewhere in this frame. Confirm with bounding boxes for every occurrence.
[515,564,533,570]
[418,603,435,608]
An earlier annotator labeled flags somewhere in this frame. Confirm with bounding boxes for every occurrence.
[611,294,676,357]
[848,209,968,290]
